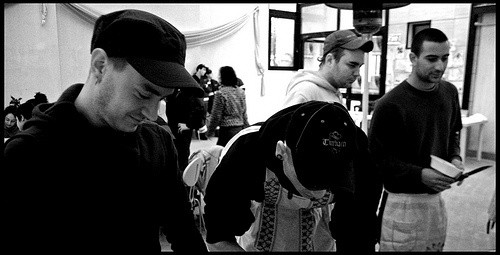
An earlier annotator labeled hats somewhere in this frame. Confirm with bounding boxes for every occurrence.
[90,10,206,98]
[287,100,354,190]
[323,30,374,56]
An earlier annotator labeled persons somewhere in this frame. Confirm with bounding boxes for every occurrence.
[282,27,375,110]
[166,63,250,197]
[203,98,381,255]
[367,26,466,255]
[2,91,49,144]
[1,7,211,254]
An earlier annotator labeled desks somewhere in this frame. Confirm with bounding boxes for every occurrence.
[349,111,488,166]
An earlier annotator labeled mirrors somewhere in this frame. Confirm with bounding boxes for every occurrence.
[268,8,297,70]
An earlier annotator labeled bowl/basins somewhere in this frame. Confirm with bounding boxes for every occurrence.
[430,154,462,179]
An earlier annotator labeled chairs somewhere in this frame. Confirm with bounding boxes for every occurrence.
[183,159,210,234]
